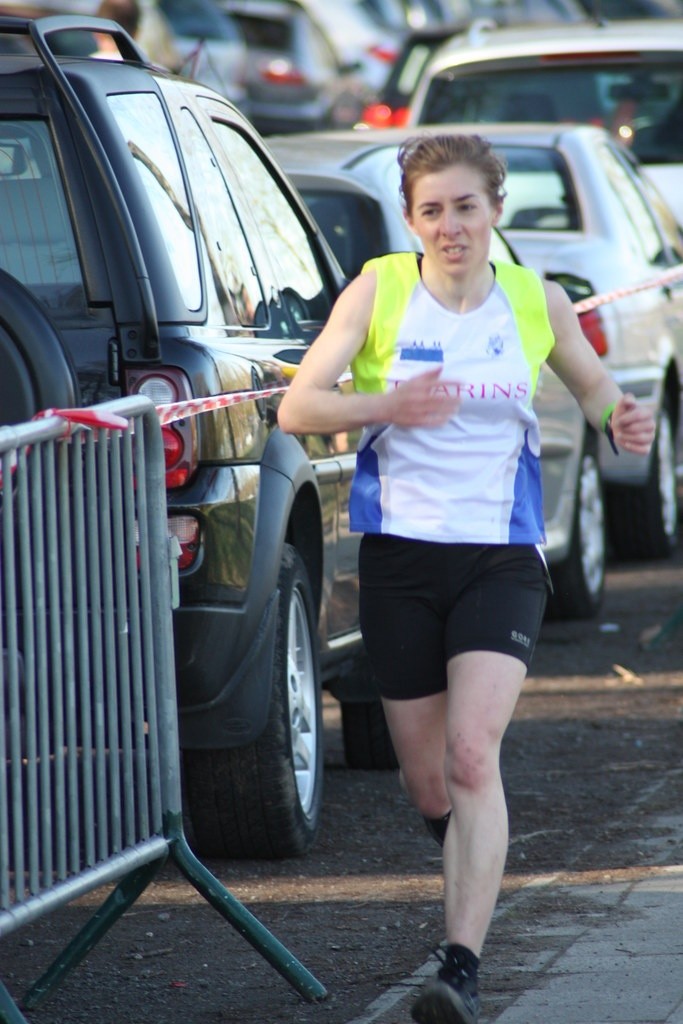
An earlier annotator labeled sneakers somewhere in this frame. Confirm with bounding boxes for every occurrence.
[411,944,482,1024]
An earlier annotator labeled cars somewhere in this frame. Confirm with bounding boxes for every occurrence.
[2,2,682,617]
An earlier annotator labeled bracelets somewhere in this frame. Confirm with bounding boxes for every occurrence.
[599,402,619,457]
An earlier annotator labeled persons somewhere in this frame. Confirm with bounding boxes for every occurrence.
[279,133,658,1024]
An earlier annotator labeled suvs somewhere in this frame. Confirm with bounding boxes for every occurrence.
[2,13,401,864]
